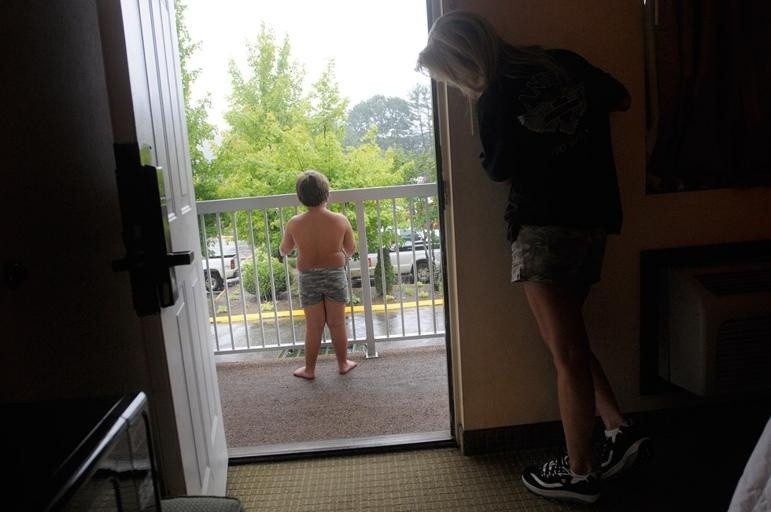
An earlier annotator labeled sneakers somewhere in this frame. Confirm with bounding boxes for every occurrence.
[523,454,603,501]
[596,427,652,480]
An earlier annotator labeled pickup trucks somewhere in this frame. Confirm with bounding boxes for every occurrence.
[200,254,240,291]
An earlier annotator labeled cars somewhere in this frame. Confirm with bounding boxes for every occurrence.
[347,227,441,286]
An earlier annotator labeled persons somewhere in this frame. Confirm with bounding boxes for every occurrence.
[279,170,358,380]
[414,10,651,503]
